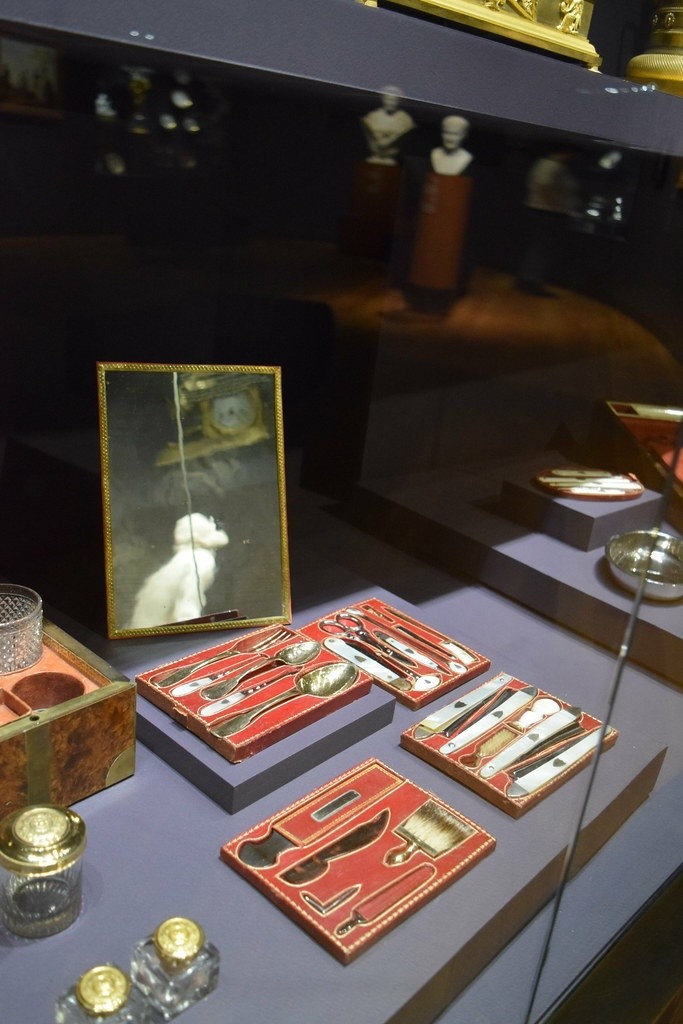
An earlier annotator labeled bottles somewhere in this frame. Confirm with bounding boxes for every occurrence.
[0,804,88,938]
[53,965,149,1024]
[130,917,221,1024]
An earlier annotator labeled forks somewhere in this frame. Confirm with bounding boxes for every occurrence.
[148,630,291,689]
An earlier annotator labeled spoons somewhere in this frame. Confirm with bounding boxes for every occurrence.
[201,641,322,700]
[210,662,358,738]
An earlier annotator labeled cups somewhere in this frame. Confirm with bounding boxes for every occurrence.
[0,583,44,676]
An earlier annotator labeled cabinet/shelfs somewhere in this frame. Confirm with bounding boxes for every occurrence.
[0,1,683,1024]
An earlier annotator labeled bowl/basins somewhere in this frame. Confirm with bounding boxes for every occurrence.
[605,530,683,600]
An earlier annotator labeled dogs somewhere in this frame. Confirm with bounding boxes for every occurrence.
[124,513,230,632]
